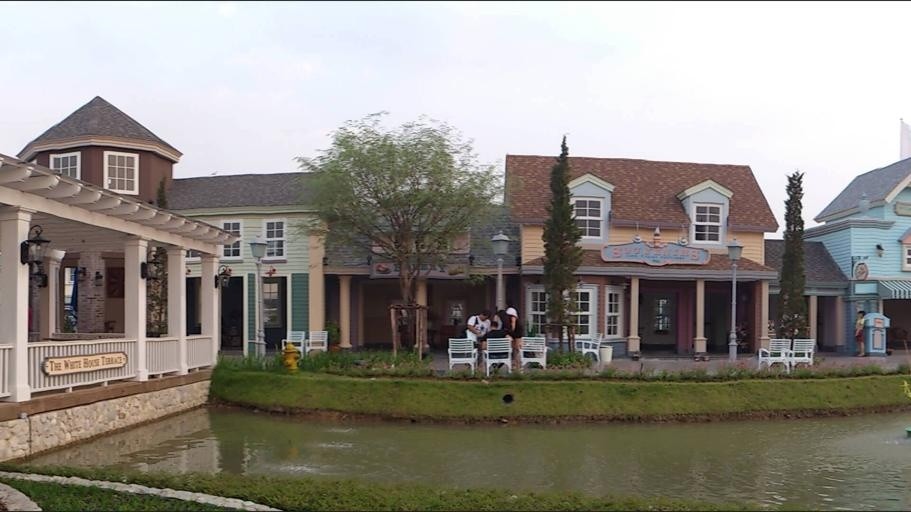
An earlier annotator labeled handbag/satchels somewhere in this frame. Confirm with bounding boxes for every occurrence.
[466,329,477,343]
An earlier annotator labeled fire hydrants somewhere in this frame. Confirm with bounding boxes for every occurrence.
[282,341,301,372]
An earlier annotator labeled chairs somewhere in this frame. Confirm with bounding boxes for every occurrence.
[758,339,816,373]
[282,331,328,358]
[448,332,604,378]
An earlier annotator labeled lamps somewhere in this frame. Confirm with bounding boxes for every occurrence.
[216,264,231,289]
[21,224,51,264]
[142,251,163,280]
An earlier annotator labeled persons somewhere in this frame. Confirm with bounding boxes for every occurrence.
[505,306,525,370]
[854,310,866,357]
[479,321,514,375]
[491,310,508,332]
[465,310,491,369]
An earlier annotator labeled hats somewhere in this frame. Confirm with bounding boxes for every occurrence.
[506,307,518,318]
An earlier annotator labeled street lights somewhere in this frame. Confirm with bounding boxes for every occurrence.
[725,235,746,365]
[484,228,510,343]
